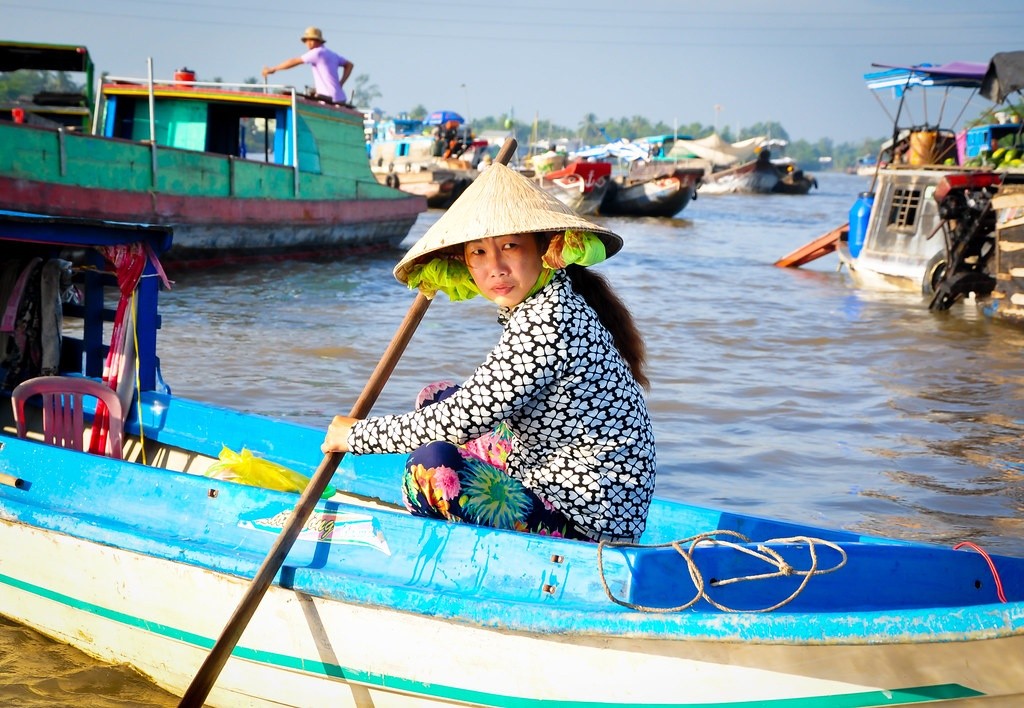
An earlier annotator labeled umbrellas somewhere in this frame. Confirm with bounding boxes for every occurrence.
[422,111,464,126]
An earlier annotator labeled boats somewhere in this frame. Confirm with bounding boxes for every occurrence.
[356,106,819,218]
[0,68,428,270]
[0,201,1023,708]
[775,43,1024,318]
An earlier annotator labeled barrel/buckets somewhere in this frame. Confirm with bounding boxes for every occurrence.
[173,66,195,88]
[848,192,875,257]
[910,131,935,165]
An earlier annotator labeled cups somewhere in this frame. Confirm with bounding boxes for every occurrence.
[12,108,24,123]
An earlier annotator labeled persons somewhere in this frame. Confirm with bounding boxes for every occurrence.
[321,160,657,544]
[262,26,354,104]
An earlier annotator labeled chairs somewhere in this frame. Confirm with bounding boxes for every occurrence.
[10,374,126,459]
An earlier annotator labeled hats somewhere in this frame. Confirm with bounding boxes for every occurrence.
[302,27,324,42]
[392,165,623,285]
